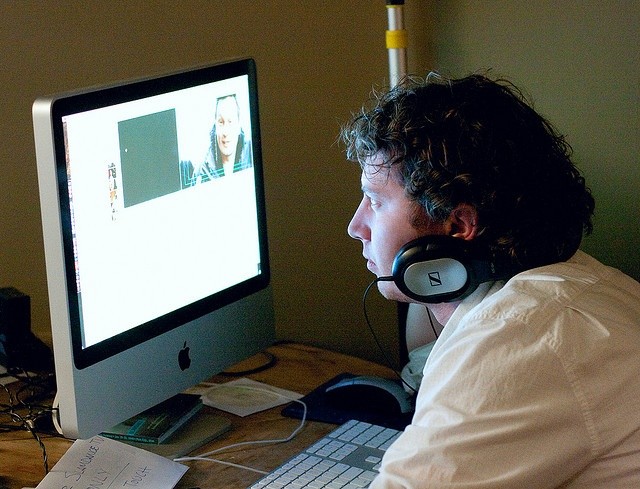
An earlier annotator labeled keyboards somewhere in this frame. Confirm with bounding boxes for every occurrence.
[242,418,404,489]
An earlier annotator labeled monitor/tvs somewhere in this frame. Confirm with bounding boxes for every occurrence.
[30,53,276,460]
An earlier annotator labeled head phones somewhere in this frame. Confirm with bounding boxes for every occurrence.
[392,225,584,304]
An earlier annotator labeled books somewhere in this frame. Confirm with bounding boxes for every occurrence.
[98,394,203,444]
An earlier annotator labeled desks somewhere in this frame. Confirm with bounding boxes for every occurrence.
[0,340,406,489]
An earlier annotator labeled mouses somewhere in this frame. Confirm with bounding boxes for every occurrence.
[326,373,411,416]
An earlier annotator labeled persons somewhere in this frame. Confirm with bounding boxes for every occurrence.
[347,76,640,489]
[190,94,253,186]
